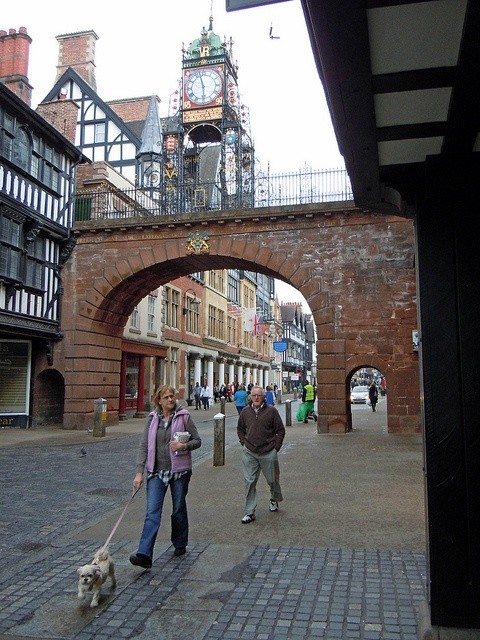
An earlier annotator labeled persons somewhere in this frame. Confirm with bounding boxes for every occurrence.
[129,384,202,569]
[369,383,378,412]
[274,384,278,397]
[193,382,202,410]
[234,385,247,415]
[200,382,211,411]
[237,386,286,523]
[213,382,232,402]
[248,382,254,390]
[283,384,287,394]
[270,383,273,389]
[236,382,246,391]
[264,386,274,406]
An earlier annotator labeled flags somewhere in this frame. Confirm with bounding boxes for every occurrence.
[252,314,261,337]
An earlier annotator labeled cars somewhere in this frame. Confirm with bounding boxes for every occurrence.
[350,386,371,404]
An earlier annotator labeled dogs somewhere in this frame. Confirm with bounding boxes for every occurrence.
[77,547,116,609]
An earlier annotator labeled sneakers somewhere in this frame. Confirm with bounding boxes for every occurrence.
[269,498,278,512]
[241,514,256,523]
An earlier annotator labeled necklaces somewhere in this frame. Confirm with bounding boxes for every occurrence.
[302,380,317,423]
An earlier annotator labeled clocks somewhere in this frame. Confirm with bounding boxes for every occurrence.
[184,69,223,105]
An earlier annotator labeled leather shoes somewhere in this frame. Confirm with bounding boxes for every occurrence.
[129,553,153,568]
[174,548,186,556]
